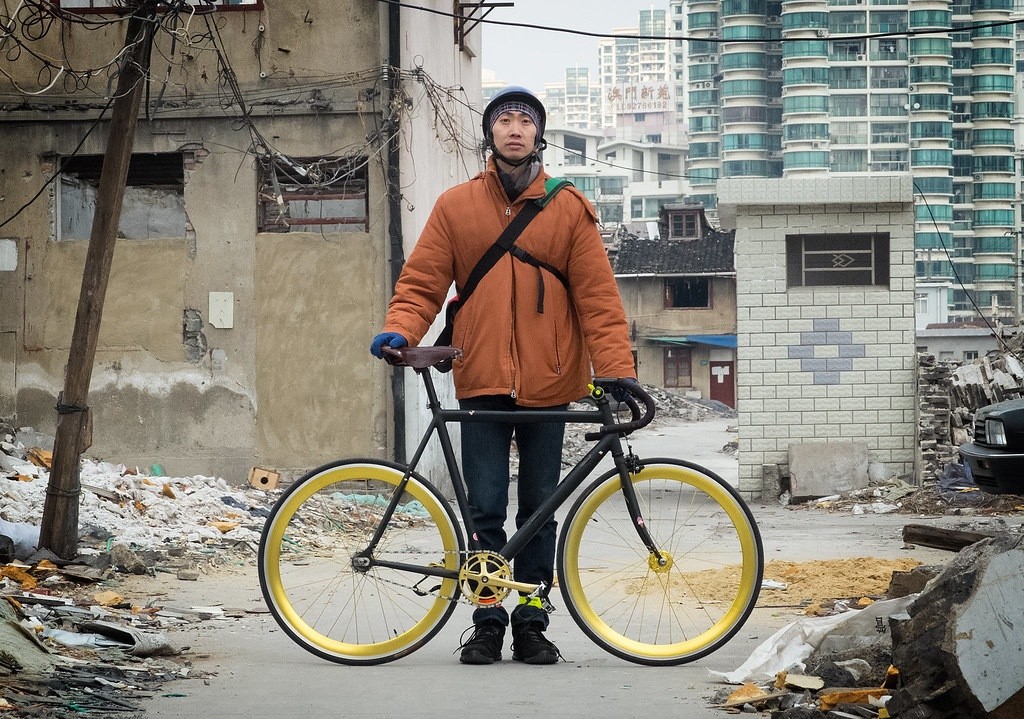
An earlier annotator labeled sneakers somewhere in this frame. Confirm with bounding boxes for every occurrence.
[459,606,509,664]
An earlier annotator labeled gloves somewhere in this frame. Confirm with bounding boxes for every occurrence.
[611,377,638,402]
[511,604,559,664]
[370,333,408,359]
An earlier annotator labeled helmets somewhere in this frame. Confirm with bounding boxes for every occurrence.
[482,85,546,139]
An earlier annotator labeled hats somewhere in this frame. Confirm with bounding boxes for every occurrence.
[490,101,541,146]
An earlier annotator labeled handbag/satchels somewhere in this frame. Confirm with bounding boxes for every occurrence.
[446,295,459,324]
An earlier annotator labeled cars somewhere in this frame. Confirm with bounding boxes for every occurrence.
[959,396,1024,498]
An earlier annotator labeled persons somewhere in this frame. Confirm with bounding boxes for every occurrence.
[369,86,655,667]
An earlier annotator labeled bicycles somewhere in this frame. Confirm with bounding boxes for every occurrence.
[255,344,767,668]
